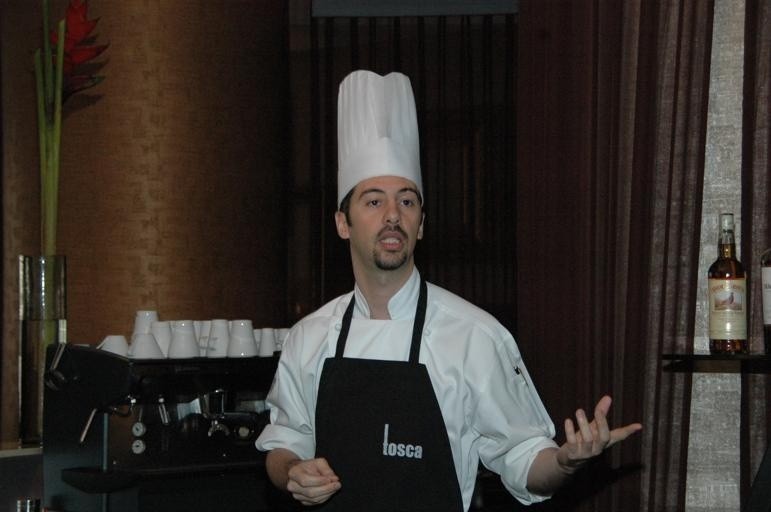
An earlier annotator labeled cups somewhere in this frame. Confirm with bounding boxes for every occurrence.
[96,310,297,360]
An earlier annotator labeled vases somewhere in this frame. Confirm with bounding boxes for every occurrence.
[17,250,67,446]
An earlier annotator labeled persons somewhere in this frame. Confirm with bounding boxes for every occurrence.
[255,176,643,512]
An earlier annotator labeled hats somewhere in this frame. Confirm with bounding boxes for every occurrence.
[337,68,427,213]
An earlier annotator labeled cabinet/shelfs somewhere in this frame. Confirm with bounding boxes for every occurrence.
[42,342,280,510]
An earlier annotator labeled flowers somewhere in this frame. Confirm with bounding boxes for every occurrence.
[32,0,108,346]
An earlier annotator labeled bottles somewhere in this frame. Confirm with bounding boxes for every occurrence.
[708,211,747,353]
[761,249,771,355]
